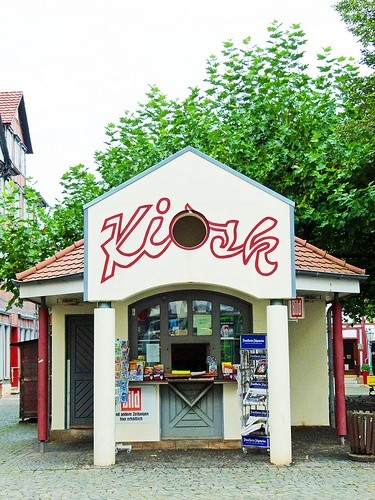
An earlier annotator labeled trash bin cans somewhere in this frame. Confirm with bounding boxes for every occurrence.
[347,408,374,458]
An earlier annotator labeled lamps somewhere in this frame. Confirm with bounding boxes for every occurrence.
[287,295,305,324]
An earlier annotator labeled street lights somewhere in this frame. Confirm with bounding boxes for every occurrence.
[366,327,375,376]
